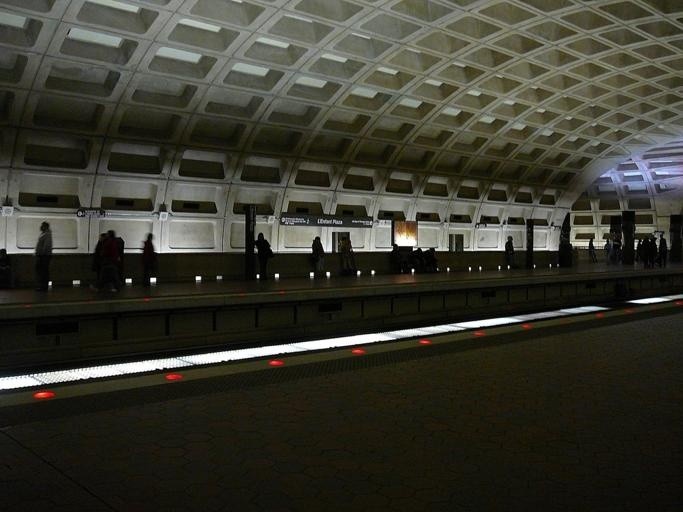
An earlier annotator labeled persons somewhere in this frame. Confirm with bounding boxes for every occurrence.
[603,234,667,269]
[339,236,353,272]
[588,237,598,264]
[311,236,325,279]
[253,232,270,279]
[87,229,125,295]
[391,243,438,274]
[32,220,53,293]
[141,233,155,286]
[504,235,516,268]
